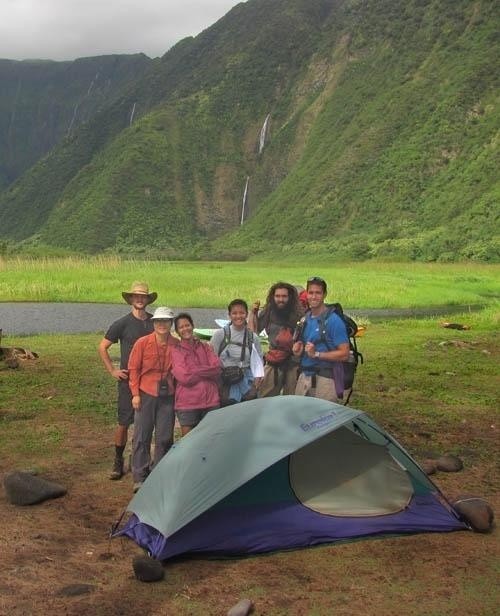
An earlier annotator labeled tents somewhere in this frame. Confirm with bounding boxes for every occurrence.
[112,392,471,562]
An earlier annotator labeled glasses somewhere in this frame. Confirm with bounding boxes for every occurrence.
[307,277,324,282]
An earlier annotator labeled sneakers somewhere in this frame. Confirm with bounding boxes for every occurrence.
[111,457,123,479]
[132,482,143,492]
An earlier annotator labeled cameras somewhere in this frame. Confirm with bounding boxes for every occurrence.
[158,384,169,397]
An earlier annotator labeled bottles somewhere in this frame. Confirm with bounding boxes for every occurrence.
[344,350,356,381]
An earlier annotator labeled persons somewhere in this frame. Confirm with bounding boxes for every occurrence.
[291,275,351,404]
[125,306,175,492]
[168,312,223,441]
[247,282,306,400]
[208,298,265,409]
[97,281,156,481]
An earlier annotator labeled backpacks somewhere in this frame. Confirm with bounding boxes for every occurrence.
[295,303,358,389]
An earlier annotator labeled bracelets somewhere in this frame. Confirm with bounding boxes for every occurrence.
[314,351,320,359]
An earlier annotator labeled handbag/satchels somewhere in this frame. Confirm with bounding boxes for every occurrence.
[222,366,243,385]
[265,349,291,367]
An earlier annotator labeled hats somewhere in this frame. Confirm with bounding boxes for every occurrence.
[122,283,157,305]
[150,307,176,319]
